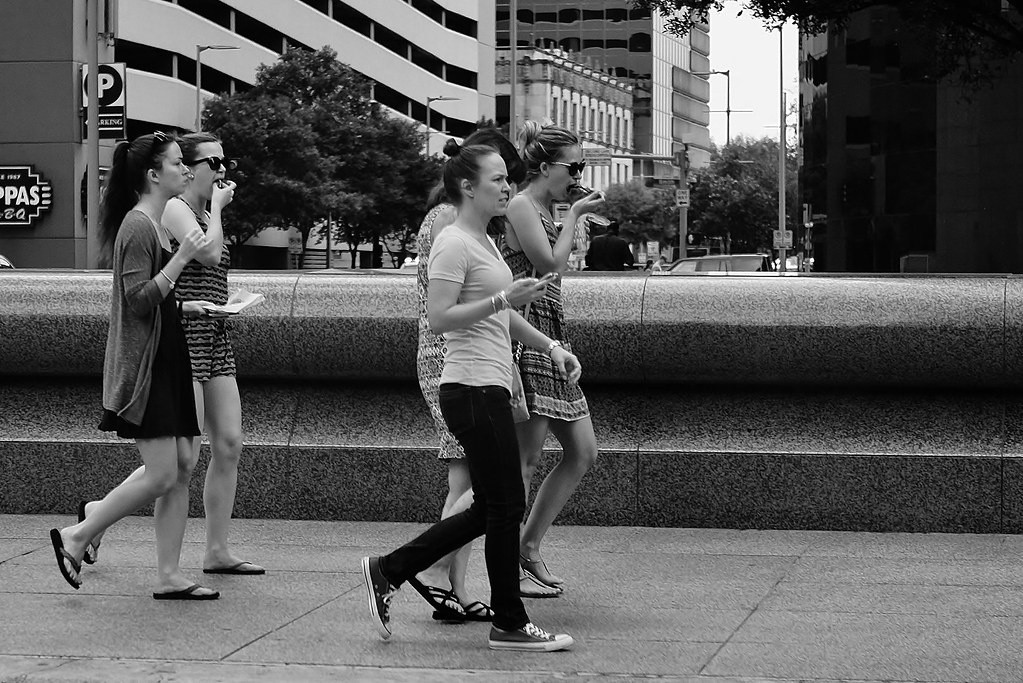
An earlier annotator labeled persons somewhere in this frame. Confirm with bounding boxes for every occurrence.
[643,260,654,272]
[584,222,635,270]
[497,120,607,599]
[361,137,581,652]
[407,128,527,623]
[49,131,265,600]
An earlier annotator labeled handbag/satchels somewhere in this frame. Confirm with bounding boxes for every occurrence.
[510,362,530,424]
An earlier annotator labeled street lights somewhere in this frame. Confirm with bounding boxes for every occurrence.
[694,70,730,144]
[426,96,461,152]
[195,44,241,135]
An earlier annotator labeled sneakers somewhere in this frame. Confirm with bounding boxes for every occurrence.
[361,556,401,639]
[487,622,574,653]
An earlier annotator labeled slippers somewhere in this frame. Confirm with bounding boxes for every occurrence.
[152,583,220,600]
[203,561,266,575]
[405,574,497,622]
[50,527,83,590]
[77,500,101,565]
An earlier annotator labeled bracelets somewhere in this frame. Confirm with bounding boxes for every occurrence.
[491,291,512,315]
[160,270,175,289]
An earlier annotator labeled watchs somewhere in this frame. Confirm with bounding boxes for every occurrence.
[545,339,562,357]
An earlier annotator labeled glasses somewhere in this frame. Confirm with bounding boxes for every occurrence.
[149,129,167,161]
[185,156,231,172]
[543,157,586,176]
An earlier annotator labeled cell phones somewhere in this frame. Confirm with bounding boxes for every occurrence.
[534,272,560,290]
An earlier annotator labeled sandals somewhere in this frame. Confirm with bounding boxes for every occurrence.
[518,551,564,599]
